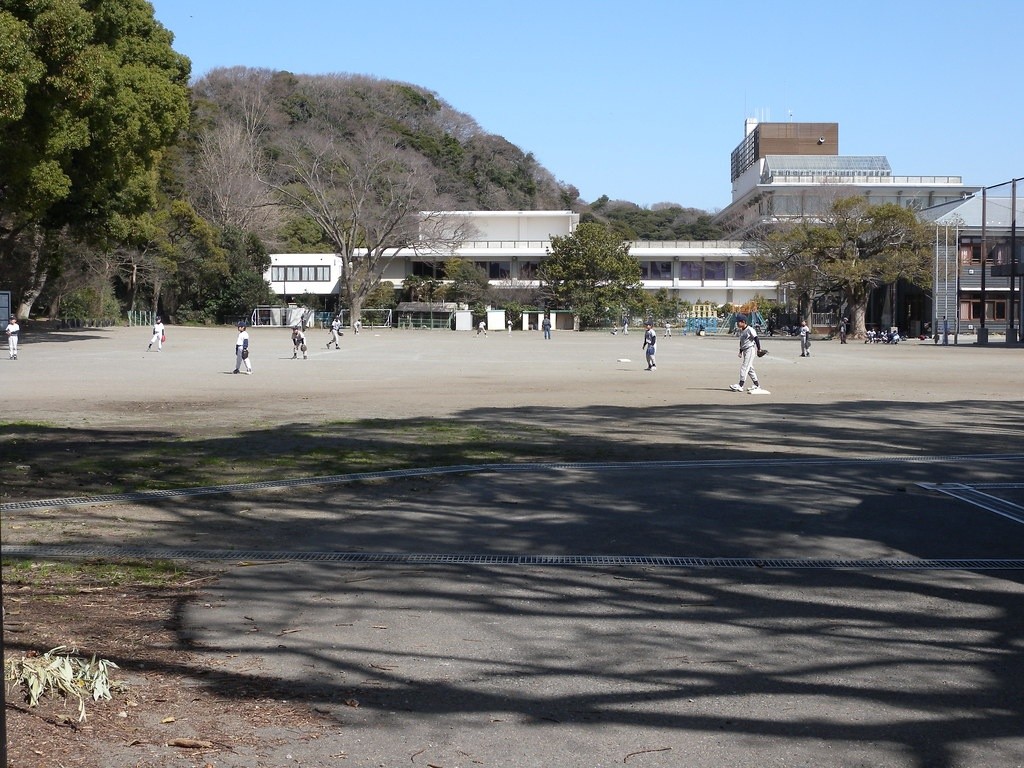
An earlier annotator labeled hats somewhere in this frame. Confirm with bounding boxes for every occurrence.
[644,321,654,326]
[734,314,748,322]
[155,316,161,320]
[9,314,16,320]
[291,325,298,330]
[235,321,246,326]
[335,315,340,319]
[844,317,849,321]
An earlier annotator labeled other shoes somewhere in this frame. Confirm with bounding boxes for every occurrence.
[729,383,744,392]
[291,356,297,360]
[747,384,762,391]
[645,365,656,371]
[233,368,240,374]
[327,343,330,349]
[304,356,307,359]
[246,369,253,374]
[336,346,340,349]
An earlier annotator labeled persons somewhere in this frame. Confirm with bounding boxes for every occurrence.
[5,314,20,360]
[233,322,254,374]
[865,327,899,344]
[476,320,488,338]
[610,323,618,335]
[326,314,343,349]
[623,317,629,335]
[729,315,761,392]
[291,325,307,359]
[664,321,671,338]
[147,316,165,352]
[542,314,552,339]
[942,316,949,344]
[353,319,361,335]
[642,320,657,371]
[507,318,513,335]
[301,310,311,333]
[800,320,811,356]
[839,317,848,344]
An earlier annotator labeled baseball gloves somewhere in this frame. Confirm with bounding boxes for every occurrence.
[338,332,344,336]
[757,349,770,358]
[301,344,307,351]
[805,341,811,348]
[242,349,249,360]
[162,335,166,342]
